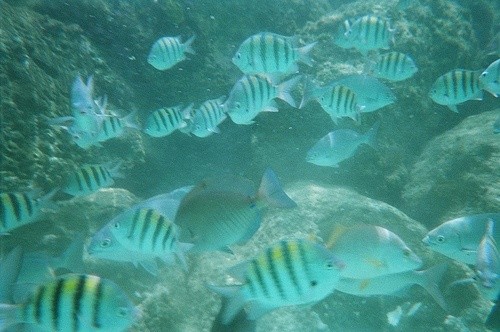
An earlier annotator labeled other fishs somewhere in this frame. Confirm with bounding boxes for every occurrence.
[0,15,500,332]
